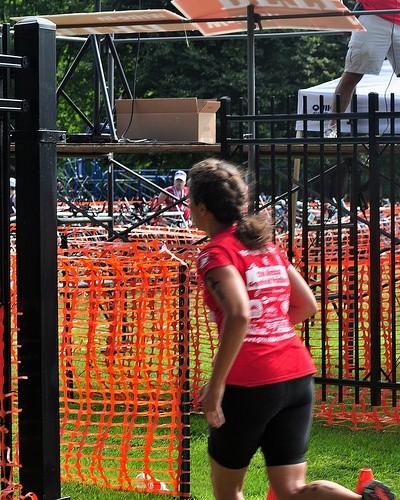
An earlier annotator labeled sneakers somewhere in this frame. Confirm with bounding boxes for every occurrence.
[361,480,395,500]
[324,123,337,139]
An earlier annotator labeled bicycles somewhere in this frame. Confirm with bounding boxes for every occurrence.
[12,192,400,284]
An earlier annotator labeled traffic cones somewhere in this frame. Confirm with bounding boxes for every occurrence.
[355,467,375,494]
[266,483,278,500]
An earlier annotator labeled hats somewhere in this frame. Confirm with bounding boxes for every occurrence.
[174,170,187,182]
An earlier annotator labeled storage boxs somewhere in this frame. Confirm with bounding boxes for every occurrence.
[114,97,221,144]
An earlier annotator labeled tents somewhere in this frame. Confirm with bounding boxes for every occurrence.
[291,59,400,246]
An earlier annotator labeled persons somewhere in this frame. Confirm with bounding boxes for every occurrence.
[153,170,192,228]
[187,158,396,500]
[323,0,400,138]
